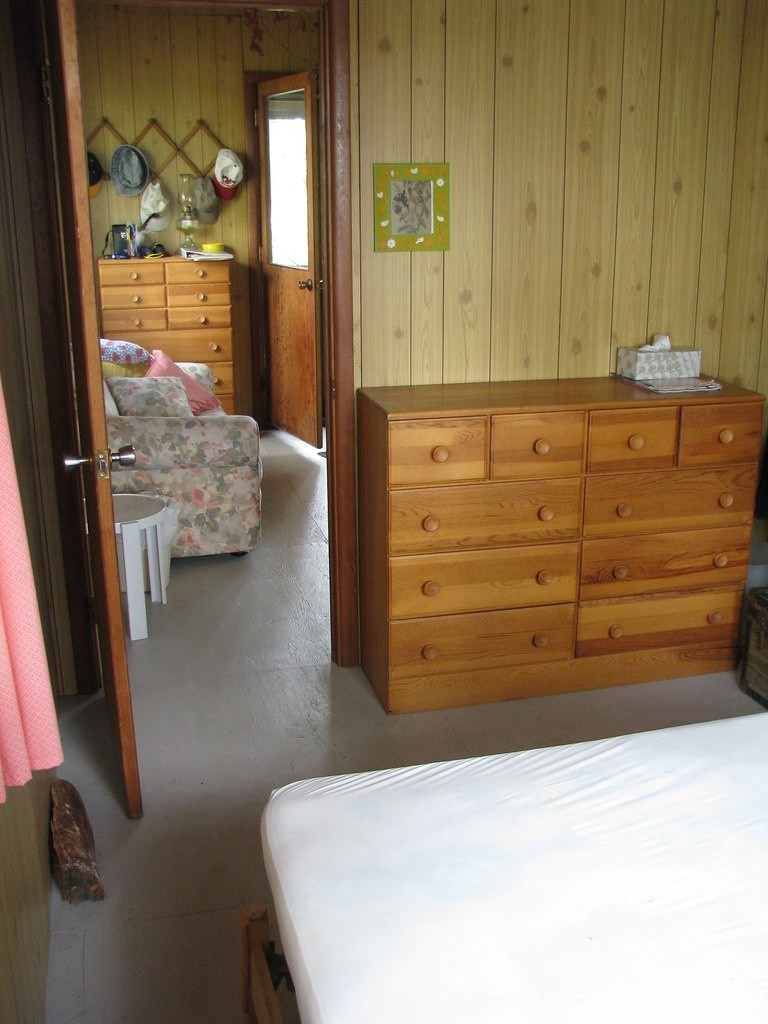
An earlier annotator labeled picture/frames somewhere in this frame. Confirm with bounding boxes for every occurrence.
[373,163,449,252]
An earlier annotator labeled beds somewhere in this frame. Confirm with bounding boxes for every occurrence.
[240,712,768,1024]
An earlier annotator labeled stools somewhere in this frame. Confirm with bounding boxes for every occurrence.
[112,494,166,641]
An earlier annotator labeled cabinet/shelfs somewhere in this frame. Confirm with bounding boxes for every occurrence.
[356,373,766,715]
[97,254,252,417]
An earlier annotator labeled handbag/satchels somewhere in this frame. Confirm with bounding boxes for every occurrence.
[112,224,128,255]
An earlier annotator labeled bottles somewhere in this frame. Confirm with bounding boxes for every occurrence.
[176,174,200,257]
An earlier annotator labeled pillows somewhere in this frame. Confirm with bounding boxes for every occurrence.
[99,339,223,417]
[99,362,263,557]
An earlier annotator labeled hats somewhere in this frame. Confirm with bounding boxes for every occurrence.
[87,151,103,197]
[193,177,221,224]
[140,175,173,231]
[110,144,149,197]
[211,148,244,200]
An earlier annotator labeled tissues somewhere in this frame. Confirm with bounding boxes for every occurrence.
[616,334,702,380]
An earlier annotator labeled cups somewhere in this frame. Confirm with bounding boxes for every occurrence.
[112,224,128,258]
